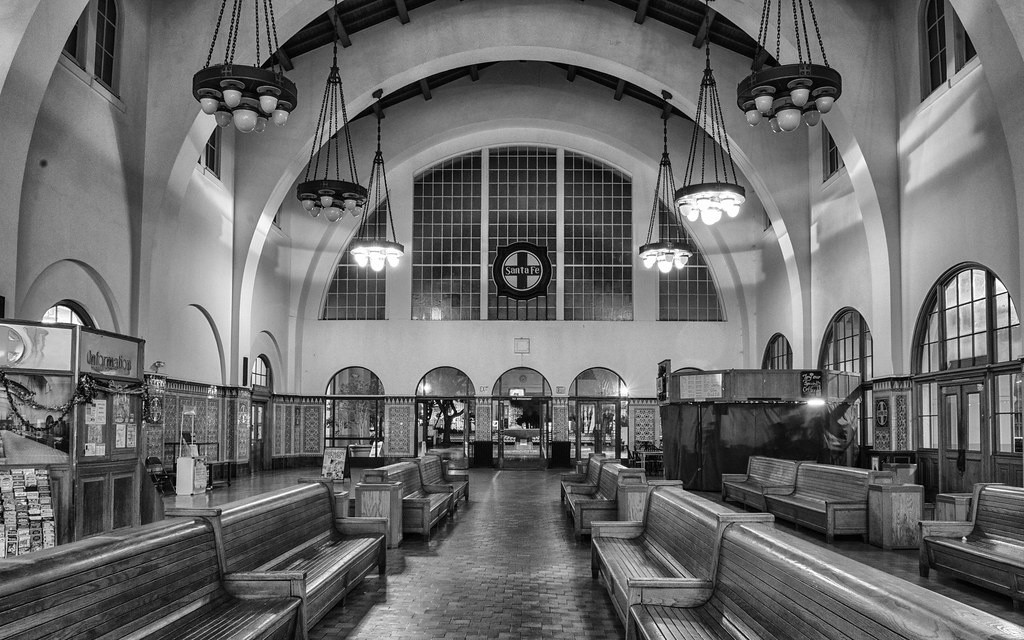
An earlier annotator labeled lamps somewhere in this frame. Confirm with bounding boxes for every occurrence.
[349,90,404,271]
[674,0,745,227]
[297,0,366,221]
[191,0,299,134]
[637,88,693,273]
[737,1,841,137]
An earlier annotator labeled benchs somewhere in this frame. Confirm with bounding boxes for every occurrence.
[410,451,470,518]
[360,457,455,542]
[627,514,1024,640]
[560,453,619,512]
[0,507,307,640]
[164,477,388,640]
[590,480,773,640]
[565,459,645,545]
[722,454,811,518]
[919,484,1024,611]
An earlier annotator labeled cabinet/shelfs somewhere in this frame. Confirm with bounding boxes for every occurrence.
[648,479,684,517]
[868,483,924,548]
[762,460,892,542]
[164,442,236,487]
[356,481,404,548]
